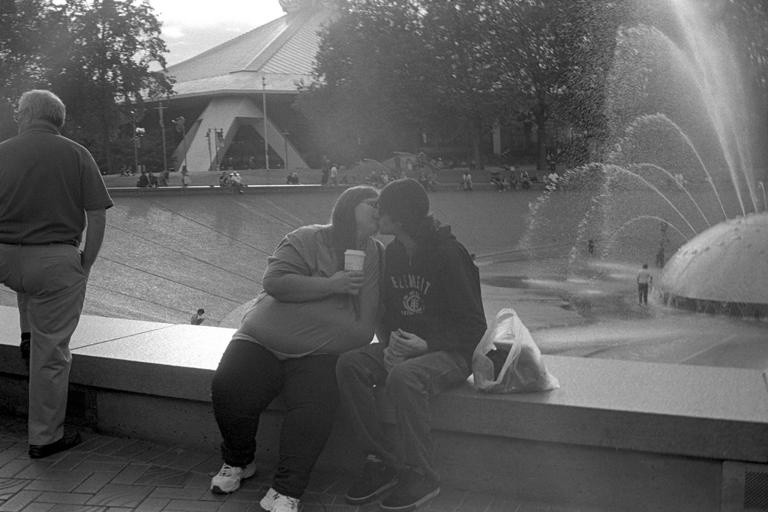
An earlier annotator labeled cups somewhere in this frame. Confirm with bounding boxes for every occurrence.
[344,249,366,273]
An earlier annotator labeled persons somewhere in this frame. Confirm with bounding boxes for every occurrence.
[328,163,338,184]
[206,181,386,512]
[178,163,191,187]
[217,170,249,195]
[118,161,175,188]
[285,170,291,184]
[319,163,328,185]
[363,145,561,193]
[0,87,116,461]
[331,174,489,511]
[635,263,653,306]
[189,307,205,326]
[290,172,298,183]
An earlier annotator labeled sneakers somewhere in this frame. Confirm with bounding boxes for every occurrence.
[345,459,440,511]
[259,488,304,512]
[210,458,257,493]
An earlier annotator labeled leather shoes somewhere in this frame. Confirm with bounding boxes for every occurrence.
[20,332,29,364]
[29,431,81,458]
